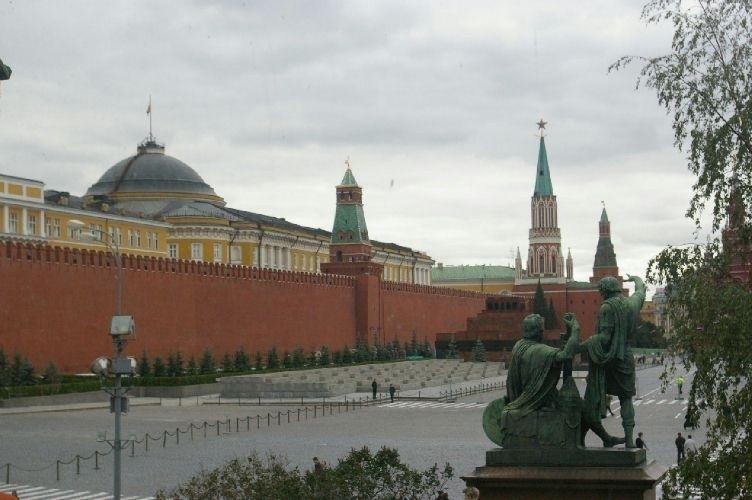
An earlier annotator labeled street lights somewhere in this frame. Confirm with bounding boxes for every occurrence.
[67,219,124,499]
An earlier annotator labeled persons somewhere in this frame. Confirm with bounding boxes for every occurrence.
[604,394,615,417]
[387,383,396,402]
[634,432,649,451]
[674,431,686,466]
[677,375,684,395]
[501,310,626,449]
[560,272,649,448]
[370,378,379,400]
[683,434,698,457]
